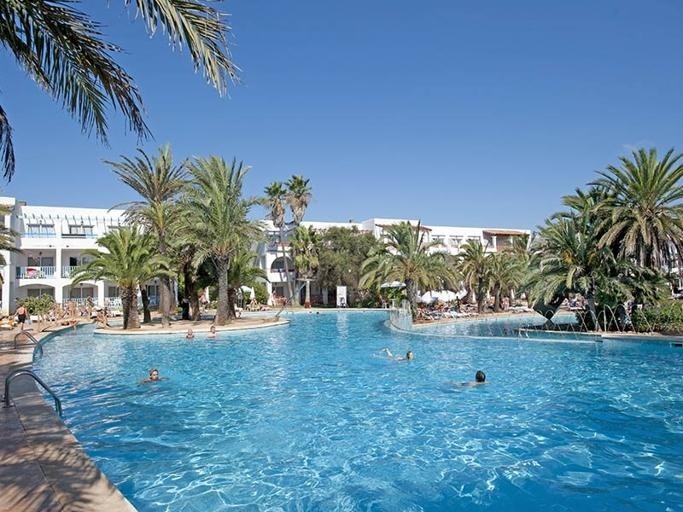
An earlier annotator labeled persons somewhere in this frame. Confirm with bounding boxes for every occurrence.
[465,371,488,386]
[208,325,218,338]
[561,293,588,307]
[416,297,508,320]
[0,295,111,333]
[251,298,256,308]
[140,368,169,384]
[197,290,209,313]
[381,348,414,361]
[186,328,195,338]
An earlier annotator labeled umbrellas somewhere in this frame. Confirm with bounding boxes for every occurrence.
[380,281,406,298]
